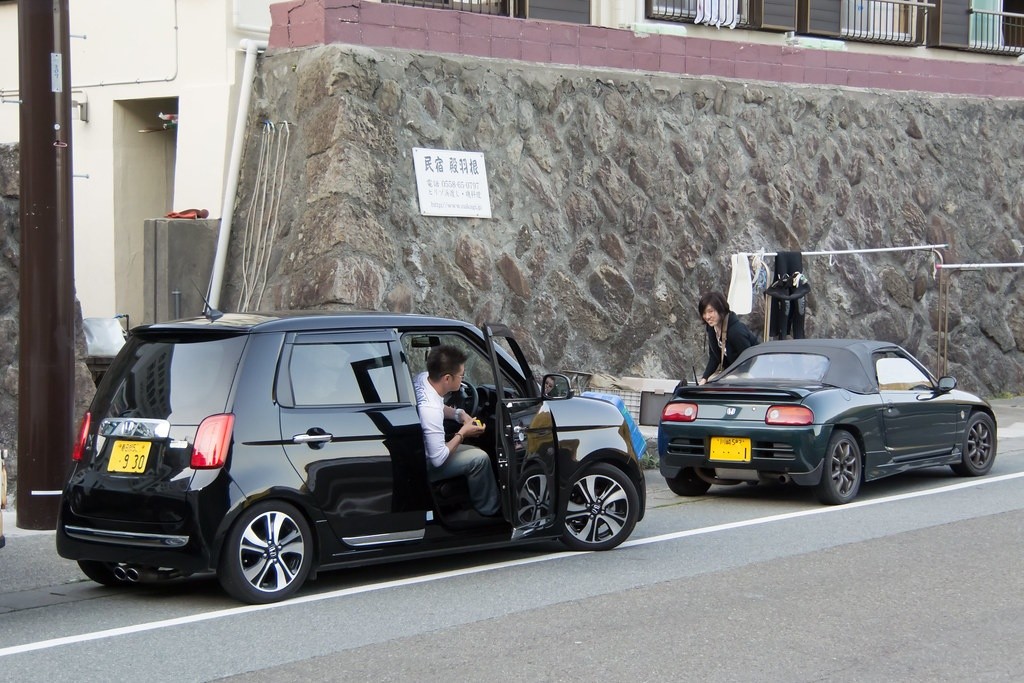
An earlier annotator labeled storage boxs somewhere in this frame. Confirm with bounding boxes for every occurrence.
[588,388,673,426]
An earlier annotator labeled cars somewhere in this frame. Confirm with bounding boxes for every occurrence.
[55,310,648,604]
[657,338,997,506]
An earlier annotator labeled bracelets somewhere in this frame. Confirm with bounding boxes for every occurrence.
[454,432,464,443]
[454,408,463,424]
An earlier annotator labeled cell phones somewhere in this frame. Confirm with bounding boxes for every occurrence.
[464,420,482,427]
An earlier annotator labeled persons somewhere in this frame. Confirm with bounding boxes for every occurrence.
[698,292,771,387]
[411,345,502,519]
[544,376,555,396]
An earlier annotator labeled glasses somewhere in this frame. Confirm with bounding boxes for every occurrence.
[451,372,465,379]
[545,381,553,389]
